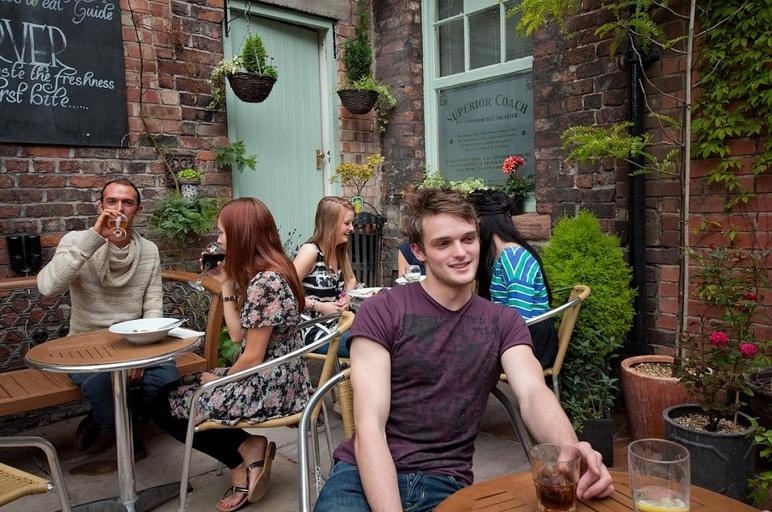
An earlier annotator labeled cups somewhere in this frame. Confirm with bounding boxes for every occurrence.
[404,265,420,283]
[529,443,582,512]
[627,437,690,512]
[108,212,129,242]
[188,241,227,292]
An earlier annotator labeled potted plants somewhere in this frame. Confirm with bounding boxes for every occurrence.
[505,0,753,447]
[337,0,396,134]
[533,208,638,464]
[329,154,385,196]
[176,168,202,198]
[417,166,485,196]
[226,34,277,104]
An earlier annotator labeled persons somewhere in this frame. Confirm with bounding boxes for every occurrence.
[396,241,426,281]
[36,179,181,462]
[466,187,560,370]
[315,188,616,511]
[292,196,358,418]
[149,197,315,512]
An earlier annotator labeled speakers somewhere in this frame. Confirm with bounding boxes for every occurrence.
[25,235,42,266]
[7,235,23,269]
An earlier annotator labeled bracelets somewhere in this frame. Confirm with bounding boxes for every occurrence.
[305,299,317,314]
[218,293,238,303]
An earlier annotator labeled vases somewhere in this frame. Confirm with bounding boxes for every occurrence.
[664,404,758,491]
[503,193,528,215]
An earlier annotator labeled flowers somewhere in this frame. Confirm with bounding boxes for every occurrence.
[676,253,762,430]
[503,156,526,192]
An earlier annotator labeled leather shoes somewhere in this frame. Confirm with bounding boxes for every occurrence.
[75,416,102,453]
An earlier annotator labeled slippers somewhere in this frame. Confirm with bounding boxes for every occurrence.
[216,483,248,512]
[245,442,276,503]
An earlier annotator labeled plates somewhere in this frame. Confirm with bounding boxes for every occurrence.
[395,275,426,285]
[348,287,390,300]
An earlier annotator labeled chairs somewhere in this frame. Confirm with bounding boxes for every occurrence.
[176,311,355,512]
[499,284,591,405]
[0,437,70,509]
[0,271,226,418]
[295,365,354,512]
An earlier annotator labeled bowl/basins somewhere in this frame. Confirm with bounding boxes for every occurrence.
[108,318,182,345]
